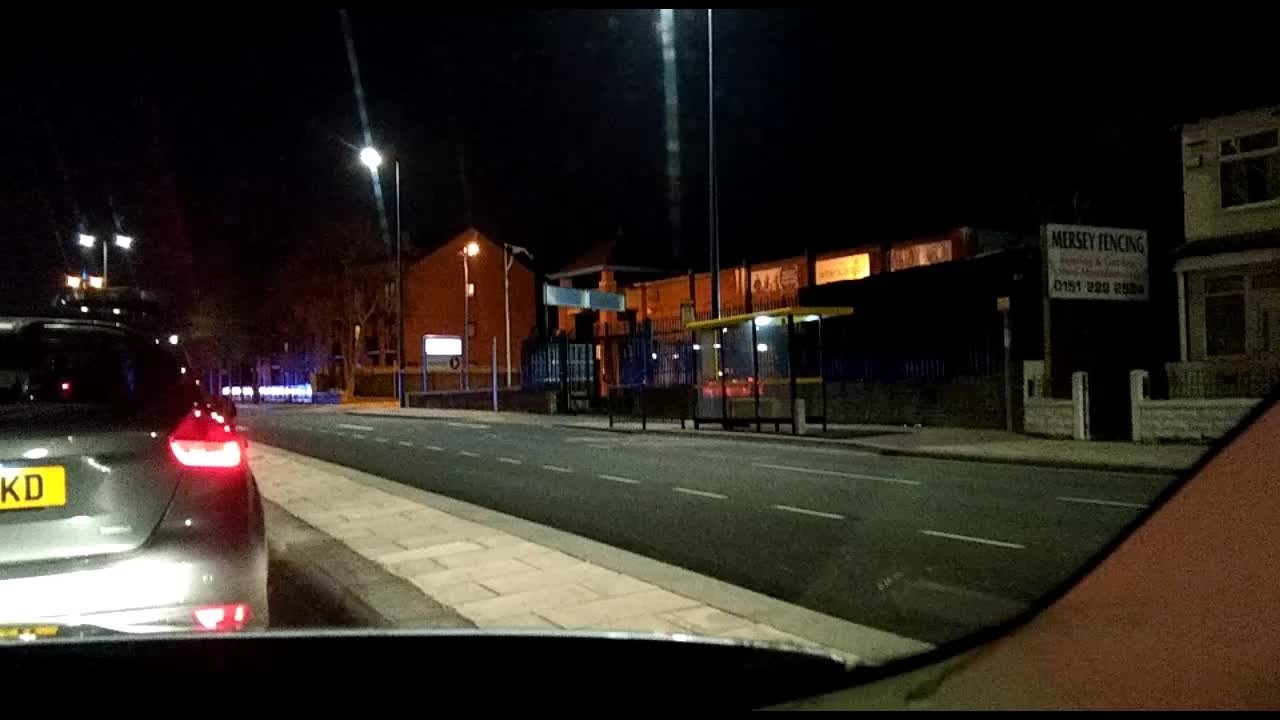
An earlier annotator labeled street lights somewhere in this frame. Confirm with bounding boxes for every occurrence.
[462,238,480,391]
[64,230,134,315]
[358,144,404,408]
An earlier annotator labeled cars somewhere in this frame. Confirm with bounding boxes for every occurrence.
[0,316,269,642]
